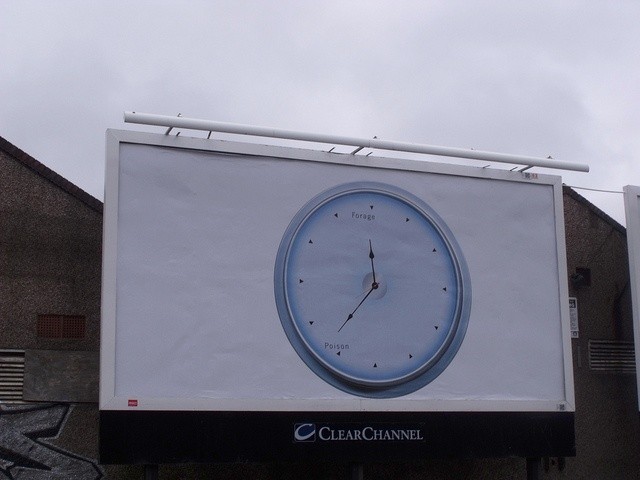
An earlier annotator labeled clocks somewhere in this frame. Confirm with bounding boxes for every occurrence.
[272,181,473,400]
